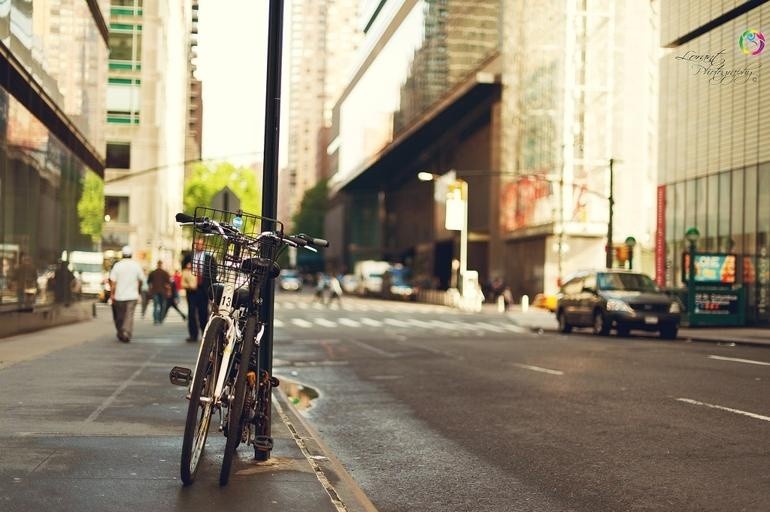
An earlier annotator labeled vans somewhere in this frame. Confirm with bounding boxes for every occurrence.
[69,250,104,300]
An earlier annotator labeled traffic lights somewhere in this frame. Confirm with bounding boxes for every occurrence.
[615,245,627,262]
[446,178,468,230]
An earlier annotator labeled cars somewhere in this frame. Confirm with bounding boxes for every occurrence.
[279,269,304,284]
[381,267,418,302]
[280,278,302,292]
[556,270,685,340]
[340,274,355,293]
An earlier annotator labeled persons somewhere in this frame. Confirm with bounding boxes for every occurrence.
[14,254,40,308]
[55,260,74,302]
[315,271,514,314]
[107,237,219,342]
[77,270,88,299]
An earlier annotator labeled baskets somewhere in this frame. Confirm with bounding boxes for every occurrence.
[190,205,283,289]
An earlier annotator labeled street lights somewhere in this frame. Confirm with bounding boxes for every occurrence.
[723,237,737,253]
[684,227,700,329]
[624,235,638,272]
[417,171,468,274]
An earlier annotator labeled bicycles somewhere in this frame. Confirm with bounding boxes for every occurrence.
[168,210,309,486]
[201,220,330,488]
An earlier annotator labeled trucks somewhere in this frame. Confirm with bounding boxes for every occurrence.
[355,260,393,296]
